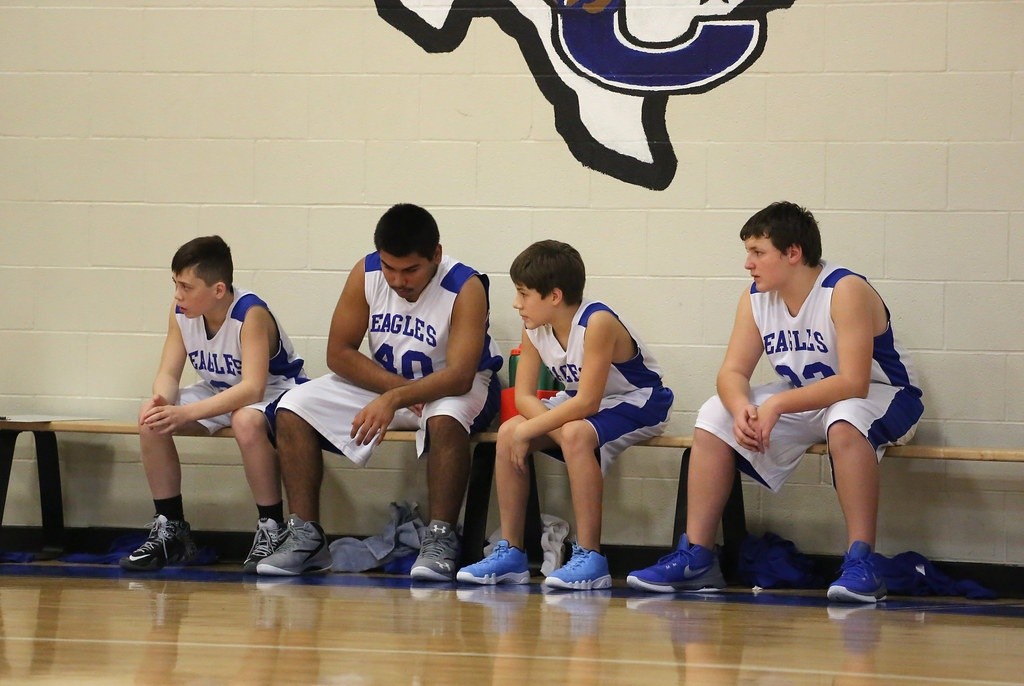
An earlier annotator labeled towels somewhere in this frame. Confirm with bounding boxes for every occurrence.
[872,551,995,599]
[315,502,428,574]
[484,511,570,577]
[733,532,810,590]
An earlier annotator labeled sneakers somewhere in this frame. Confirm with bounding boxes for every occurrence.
[410,519,458,582]
[627,533,727,592]
[256,515,334,574]
[456,539,530,585]
[119,512,199,572]
[545,545,612,589]
[827,542,887,603]
[243,513,286,575]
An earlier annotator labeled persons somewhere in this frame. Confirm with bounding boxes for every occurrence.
[626,202,924,602]
[457,240,674,589]
[119,236,311,572]
[256,203,509,579]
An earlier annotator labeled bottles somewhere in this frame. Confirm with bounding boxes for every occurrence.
[554,377,564,391]
[540,361,553,390]
[509,350,521,387]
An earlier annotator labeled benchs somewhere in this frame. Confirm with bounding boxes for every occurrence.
[0,418,1024,579]
